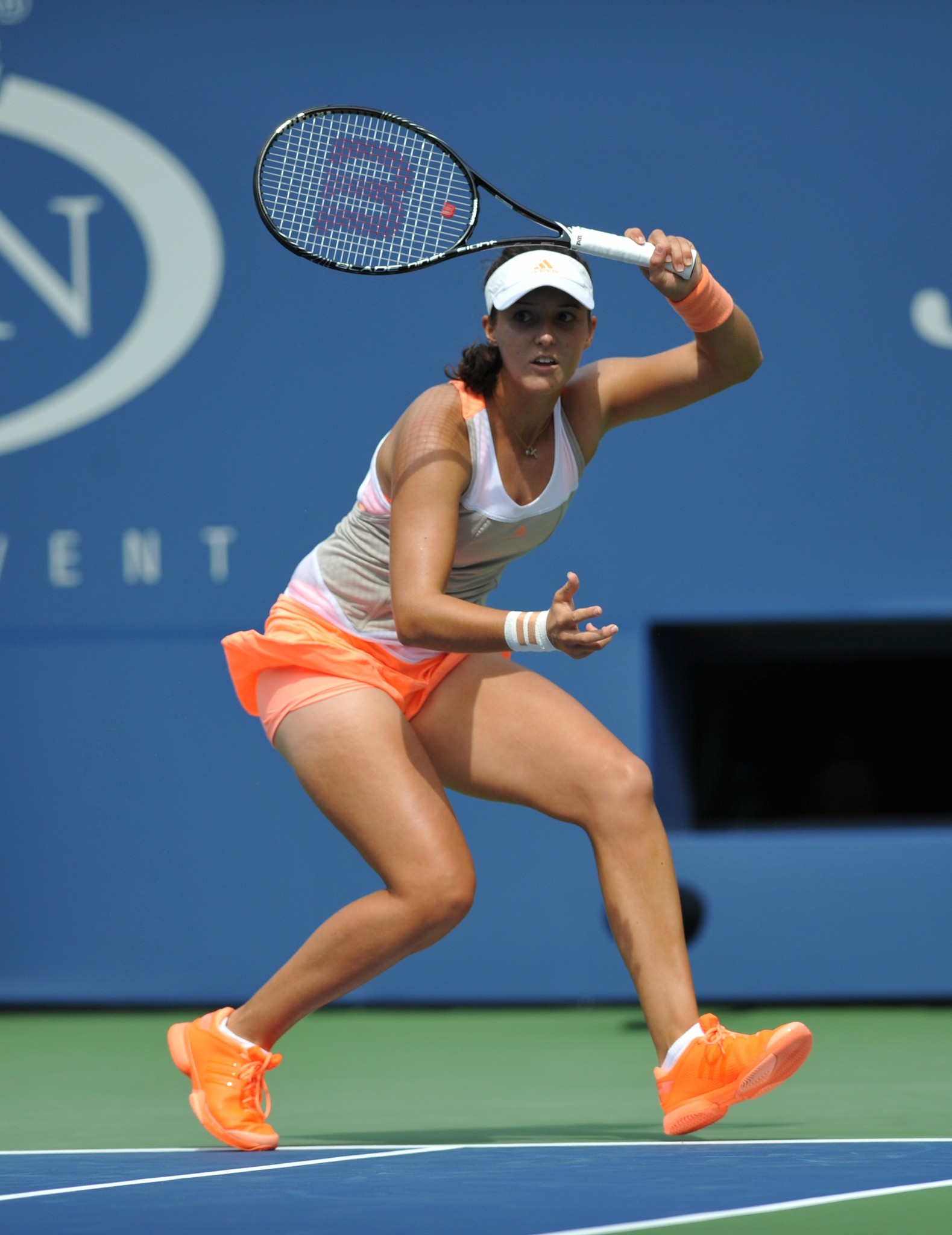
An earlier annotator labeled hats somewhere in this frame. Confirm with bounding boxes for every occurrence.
[484,247,596,317]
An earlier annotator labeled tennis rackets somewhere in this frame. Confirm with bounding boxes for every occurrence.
[250,102,699,281]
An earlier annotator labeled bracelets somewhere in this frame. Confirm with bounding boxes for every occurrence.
[667,264,734,331]
[504,608,559,652]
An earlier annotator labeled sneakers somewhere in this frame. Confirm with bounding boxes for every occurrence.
[654,1012,814,1136]
[166,1002,282,1154]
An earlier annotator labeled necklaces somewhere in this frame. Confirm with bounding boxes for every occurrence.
[494,392,537,459]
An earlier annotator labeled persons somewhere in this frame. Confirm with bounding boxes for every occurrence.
[168,226,814,1161]
